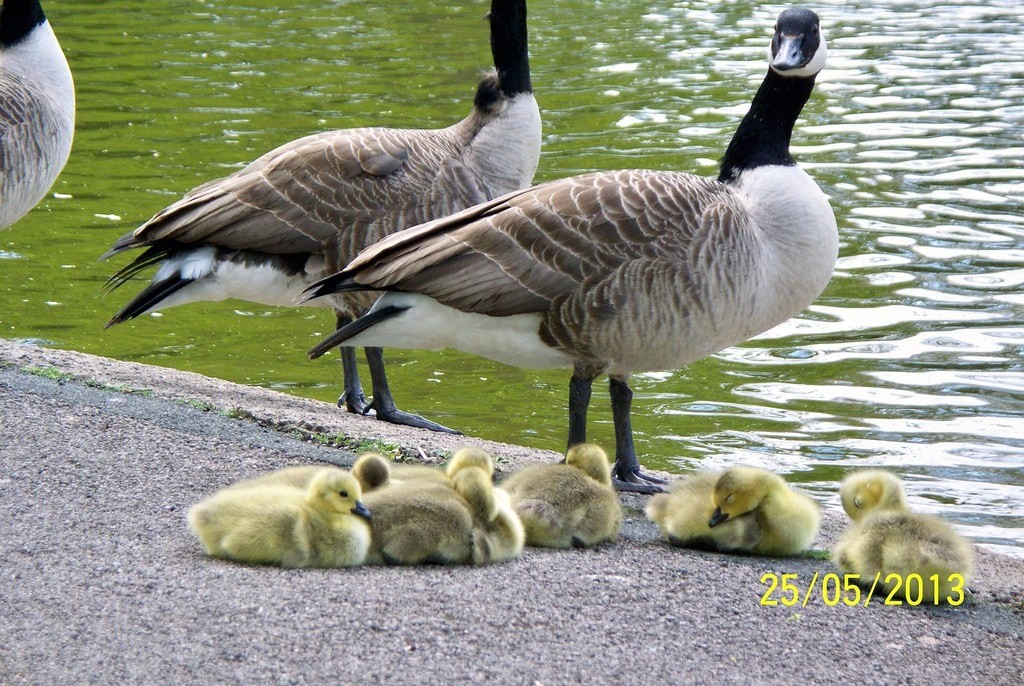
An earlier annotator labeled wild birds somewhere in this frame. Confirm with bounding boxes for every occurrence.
[185,452,391,568]
[101,0,544,434]
[292,2,839,492]
[502,441,622,549]
[645,466,822,560]
[0,0,77,233]
[372,447,527,566]
[829,468,970,605]
